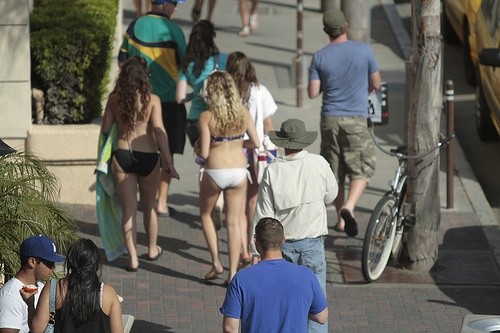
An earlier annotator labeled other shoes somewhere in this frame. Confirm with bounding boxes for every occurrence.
[249,16,257,28]
[238,26,249,36]
[211,205,222,230]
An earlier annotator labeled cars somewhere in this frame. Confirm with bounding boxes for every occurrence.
[440,0,500,142]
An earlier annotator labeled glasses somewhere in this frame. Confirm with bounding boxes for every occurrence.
[134,55,147,67]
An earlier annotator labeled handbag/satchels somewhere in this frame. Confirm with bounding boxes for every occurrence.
[44,277,56,333]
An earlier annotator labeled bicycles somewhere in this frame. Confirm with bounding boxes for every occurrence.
[361,121,457,283]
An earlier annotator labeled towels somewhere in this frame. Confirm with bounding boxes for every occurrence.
[95,115,137,261]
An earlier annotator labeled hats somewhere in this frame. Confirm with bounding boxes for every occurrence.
[323,9,345,32]
[268,119,318,149]
[19,235,66,262]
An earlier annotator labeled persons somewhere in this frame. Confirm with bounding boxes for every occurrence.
[191,0,216,25]
[102,56,180,269]
[219,218,330,333]
[308,8,382,236]
[251,119,339,333]
[175,19,232,229]
[19,238,124,333]
[193,70,260,286]
[0,235,66,333]
[118,0,187,170]
[222,51,277,268]
[132,0,152,18]
[238,0,258,36]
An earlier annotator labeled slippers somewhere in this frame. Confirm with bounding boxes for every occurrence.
[340,208,358,237]
[203,271,224,280]
[159,205,176,217]
[335,223,344,231]
[127,261,140,272]
[239,257,251,269]
[148,246,163,261]
[224,281,229,286]
